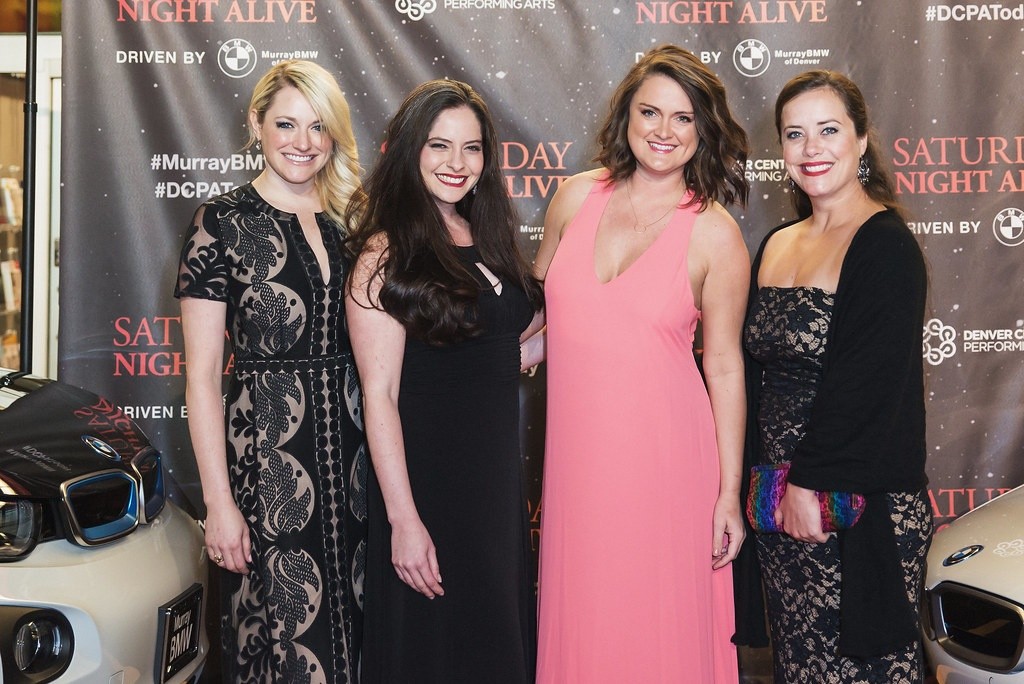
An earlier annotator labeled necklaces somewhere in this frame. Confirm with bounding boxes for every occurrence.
[626,179,679,233]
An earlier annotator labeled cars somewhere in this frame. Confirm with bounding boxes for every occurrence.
[920,480,1023,684]
[0,364,210,684]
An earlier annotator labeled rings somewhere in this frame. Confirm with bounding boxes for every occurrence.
[214,555,223,563]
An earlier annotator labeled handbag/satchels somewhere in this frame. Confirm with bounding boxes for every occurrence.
[746,462,866,535]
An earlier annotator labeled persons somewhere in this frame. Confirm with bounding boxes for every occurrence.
[174,59,367,684]
[741,71,935,684]
[519,45,751,684]
[346,80,546,684]
